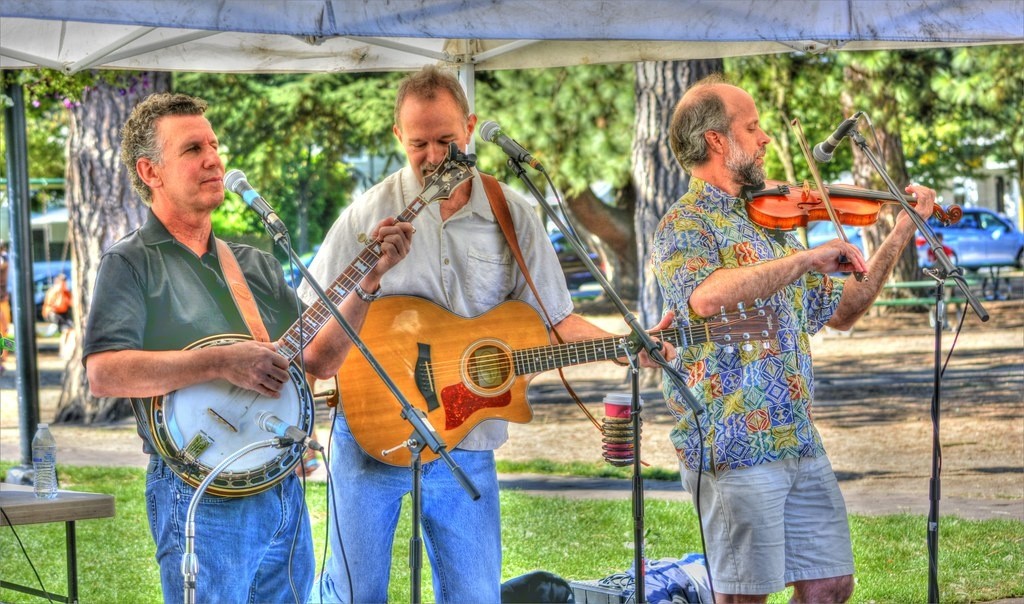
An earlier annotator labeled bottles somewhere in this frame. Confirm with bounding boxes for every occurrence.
[30,422,58,501]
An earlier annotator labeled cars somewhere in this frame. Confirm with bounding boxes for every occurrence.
[545,219,606,291]
[909,204,1024,281]
[805,220,869,280]
[4,258,72,326]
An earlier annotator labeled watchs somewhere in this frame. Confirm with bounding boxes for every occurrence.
[355,283,382,302]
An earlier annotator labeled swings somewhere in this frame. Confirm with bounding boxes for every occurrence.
[47,226,72,325]
[34,183,60,335]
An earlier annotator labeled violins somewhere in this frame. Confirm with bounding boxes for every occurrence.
[747,178,963,231]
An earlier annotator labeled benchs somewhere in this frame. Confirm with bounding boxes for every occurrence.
[872,298,969,327]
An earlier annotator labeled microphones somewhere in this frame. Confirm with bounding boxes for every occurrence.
[813,110,862,163]
[223,169,288,237]
[479,121,544,172]
[255,411,324,452]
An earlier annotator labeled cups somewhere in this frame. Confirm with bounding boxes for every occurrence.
[601,392,646,467]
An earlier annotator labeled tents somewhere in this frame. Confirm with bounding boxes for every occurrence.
[0,0,1024,171]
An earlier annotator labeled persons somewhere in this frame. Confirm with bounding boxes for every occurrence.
[651,80,937,604]
[927,232,955,331]
[294,67,675,604]
[81,91,414,604]
[41,274,74,328]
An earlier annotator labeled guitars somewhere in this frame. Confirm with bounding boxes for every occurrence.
[332,290,782,470]
[147,140,479,501]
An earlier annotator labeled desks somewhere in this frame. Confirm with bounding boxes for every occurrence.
[883,280,959,326]
[0,491,115,603]
